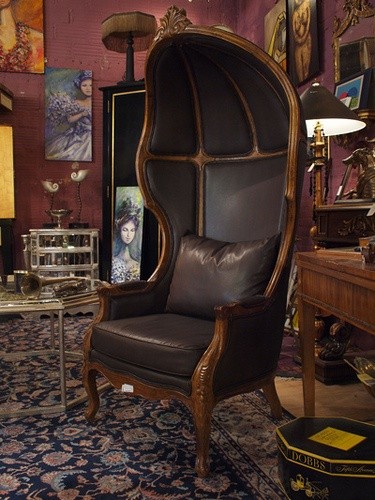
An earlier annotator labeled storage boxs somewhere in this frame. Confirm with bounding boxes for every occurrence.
[274,415,375,500]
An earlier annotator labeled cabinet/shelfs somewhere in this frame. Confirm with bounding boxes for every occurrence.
[97,85,165,284]
[20,228,100,280]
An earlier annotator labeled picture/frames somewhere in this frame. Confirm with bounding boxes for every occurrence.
[334,67,374,112]
[110,184,144,286]
[263,0,322,88]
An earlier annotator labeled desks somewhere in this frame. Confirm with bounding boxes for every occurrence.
[0,276,115,418]
[294,247,375,418]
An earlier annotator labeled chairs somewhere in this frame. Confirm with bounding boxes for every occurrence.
[80,3,308,479]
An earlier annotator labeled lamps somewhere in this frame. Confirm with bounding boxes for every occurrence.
[69,169,89,229]
[101,10,158,86]
[42,179,60,228]
[298,81,367,252]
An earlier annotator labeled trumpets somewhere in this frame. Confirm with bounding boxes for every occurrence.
[21,272,107,298]
